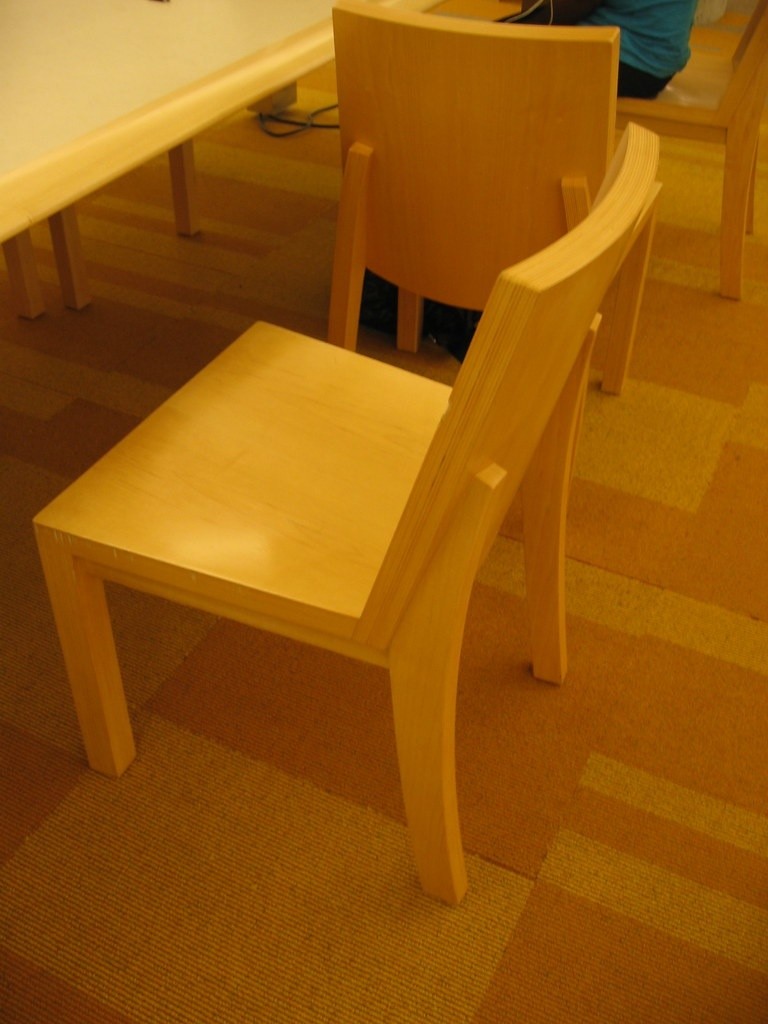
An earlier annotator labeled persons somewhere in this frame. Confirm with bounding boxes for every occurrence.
[362,0,695,351]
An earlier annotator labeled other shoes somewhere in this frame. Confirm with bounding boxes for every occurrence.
[358,271,483,364]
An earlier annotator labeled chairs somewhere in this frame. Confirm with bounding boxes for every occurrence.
[32,122,667,908]
[324,0,661,516]
[617,0,768,300]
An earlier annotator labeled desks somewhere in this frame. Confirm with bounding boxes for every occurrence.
[1,0,438,245]
